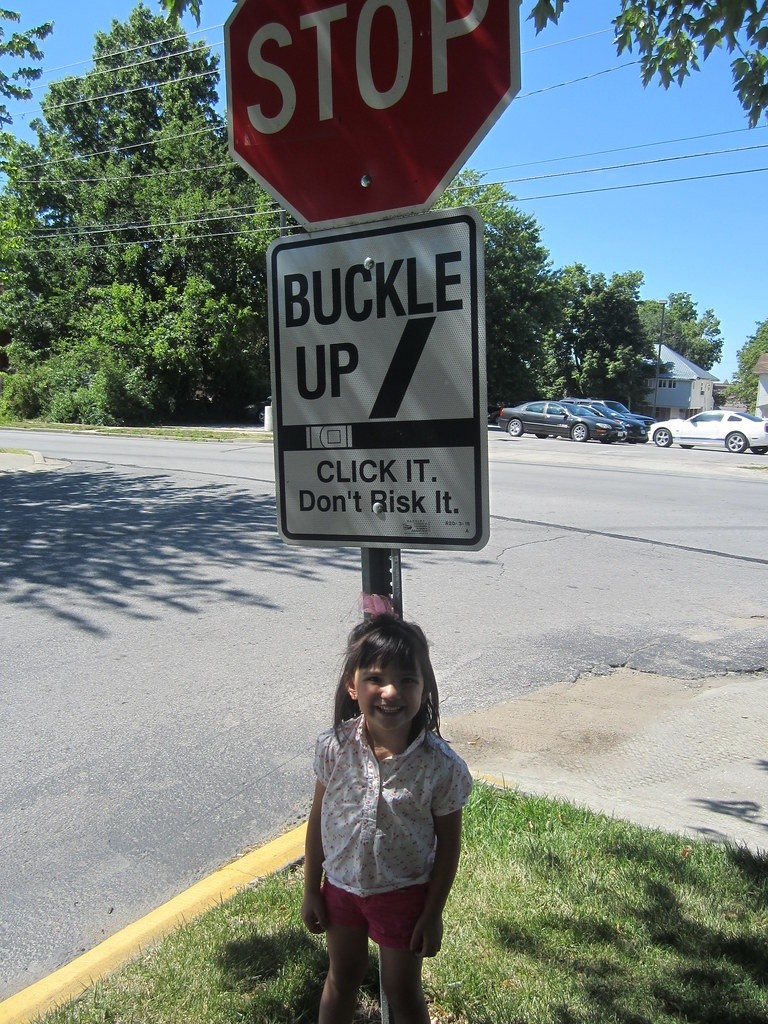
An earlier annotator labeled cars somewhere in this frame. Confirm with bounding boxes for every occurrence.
[487,403,503,424]
[243,399,271,424]
[497,401,627,443]
[646,410,768,455]
[576,404,649,446]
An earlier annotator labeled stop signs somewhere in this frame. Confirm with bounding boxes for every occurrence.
[220,1,522,232]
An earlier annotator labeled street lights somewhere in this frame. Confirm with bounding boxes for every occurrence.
[651,300,667,420]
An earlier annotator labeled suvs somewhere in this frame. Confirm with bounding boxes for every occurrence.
[560,397,657,432]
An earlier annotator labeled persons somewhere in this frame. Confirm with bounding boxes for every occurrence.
[300,593,472,1024]
